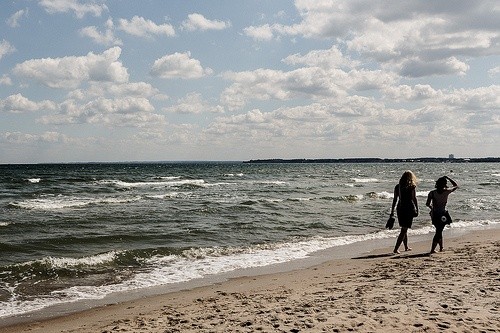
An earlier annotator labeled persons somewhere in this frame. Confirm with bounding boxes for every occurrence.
[389,170,418,253]
[426,175,458,253]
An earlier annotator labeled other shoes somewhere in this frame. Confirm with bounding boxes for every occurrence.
[385,217,395,230]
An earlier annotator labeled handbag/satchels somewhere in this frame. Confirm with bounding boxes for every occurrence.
[432,209,454,226]
